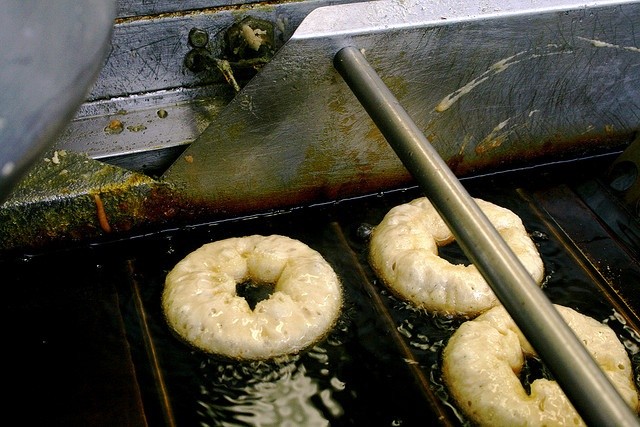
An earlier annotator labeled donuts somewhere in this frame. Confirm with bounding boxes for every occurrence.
[372,194,544,320]
[160,234,345,361]
[443,304,637,427]
